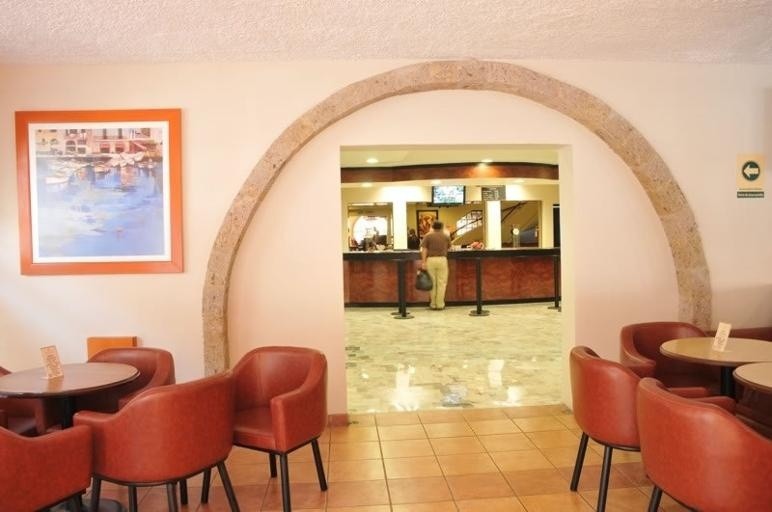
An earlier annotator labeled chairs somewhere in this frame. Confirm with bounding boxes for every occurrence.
[568,321,772,512]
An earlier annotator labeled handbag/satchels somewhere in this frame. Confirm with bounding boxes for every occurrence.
[415,269,433,290]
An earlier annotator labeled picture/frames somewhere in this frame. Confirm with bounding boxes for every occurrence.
[14,107,186,277]
[417,210,439,240]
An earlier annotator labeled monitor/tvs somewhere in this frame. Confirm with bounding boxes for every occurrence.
[432,186,465,205]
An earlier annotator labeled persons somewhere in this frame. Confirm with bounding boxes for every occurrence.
[420,223,452,310]
[407,227,419,249]
